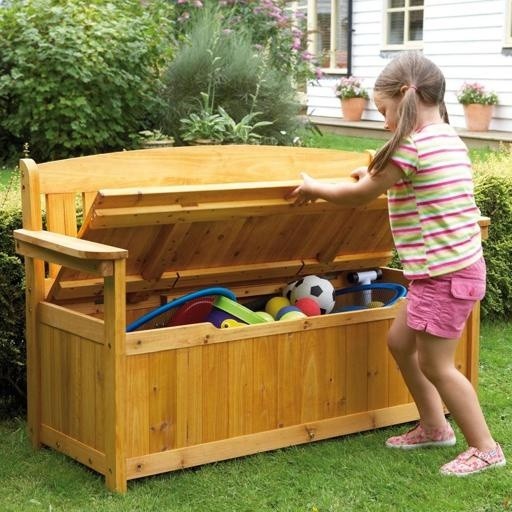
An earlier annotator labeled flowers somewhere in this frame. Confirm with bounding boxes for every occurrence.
[456,80,497,105]
[332,72,371,100]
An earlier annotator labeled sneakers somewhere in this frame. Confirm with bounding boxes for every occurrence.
[383,420,457,451]
[438,441,507,478]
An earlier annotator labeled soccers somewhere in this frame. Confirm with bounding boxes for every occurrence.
[289,273,336,315]
[282,280,298,301]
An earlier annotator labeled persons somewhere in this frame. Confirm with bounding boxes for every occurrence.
[281,50,507,479]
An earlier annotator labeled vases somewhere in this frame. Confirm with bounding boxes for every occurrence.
[341,95,366,121]
[463,102,492,131]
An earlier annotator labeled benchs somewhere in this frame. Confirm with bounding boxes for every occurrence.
[17,142,495,494]
